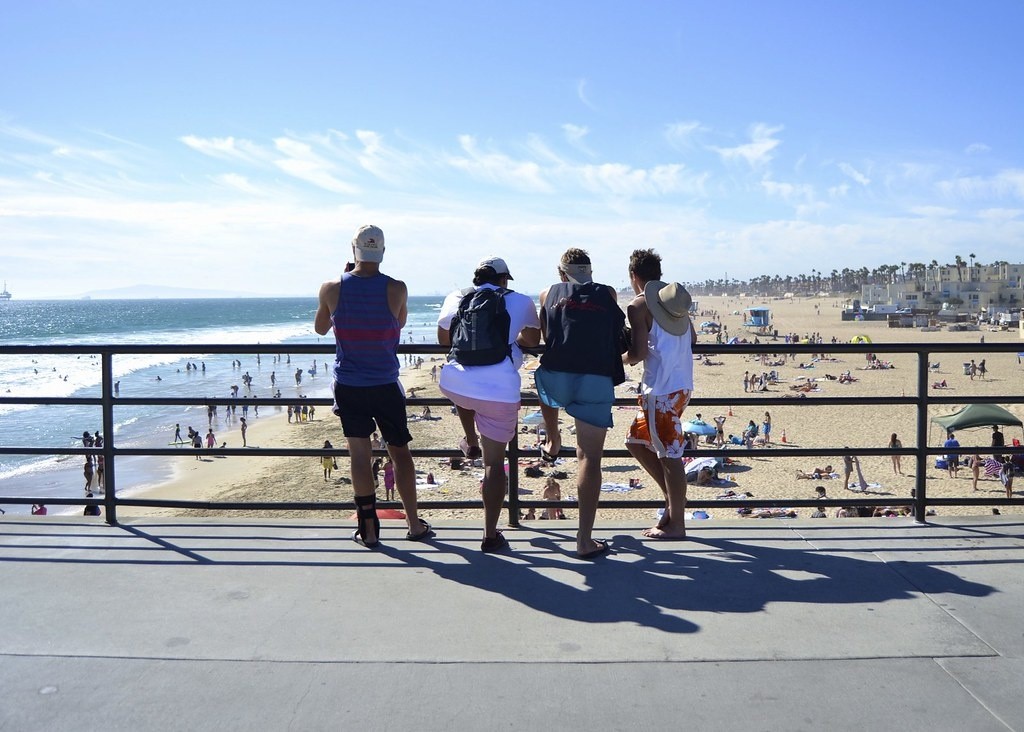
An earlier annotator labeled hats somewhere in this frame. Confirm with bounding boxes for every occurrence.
[352,224,384,263]
[478,254,514,281]
[949,434,955,437]
[559,261,592,285]
[992,425,999,429]
[644,280,692,336]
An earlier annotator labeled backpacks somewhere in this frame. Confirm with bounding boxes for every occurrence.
[449,287,514,366]
[560,283,611,356]
[427,473,434,484]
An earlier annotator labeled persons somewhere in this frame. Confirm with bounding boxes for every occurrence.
[942,425,1015,498]
[622,248,697,538]
[693,314,894,398]
[114,381,120,393]
[155,354,328,460]
[314,225,432,548]
[967,359,988,379]
[0,431,104,516]
[436,253,542,553]
[534,248,626,560]
[888,433,903,476]
[682,411,771,486]
[741,446,1001,518]
[403,336,444,382]
[320,391,563,520]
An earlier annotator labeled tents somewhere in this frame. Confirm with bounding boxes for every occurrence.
[928,404,1024,447]
[851,335,872,343]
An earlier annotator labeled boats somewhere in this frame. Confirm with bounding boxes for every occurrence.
[0,280,12,300]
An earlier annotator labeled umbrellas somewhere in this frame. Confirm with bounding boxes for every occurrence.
[522,412,546,425]
[682,419,717,435]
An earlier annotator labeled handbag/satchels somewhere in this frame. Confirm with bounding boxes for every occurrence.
[524,463,568,479]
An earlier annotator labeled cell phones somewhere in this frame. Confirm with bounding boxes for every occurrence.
[347,263,355,272]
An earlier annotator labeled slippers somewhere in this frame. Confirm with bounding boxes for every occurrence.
[406,518,431,540]
[352,529,380,547]
[538,441,558,462]
[579,538,609,559]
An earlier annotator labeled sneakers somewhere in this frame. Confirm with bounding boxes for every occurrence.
[459,435,481,459]
[481,531,509,553]
